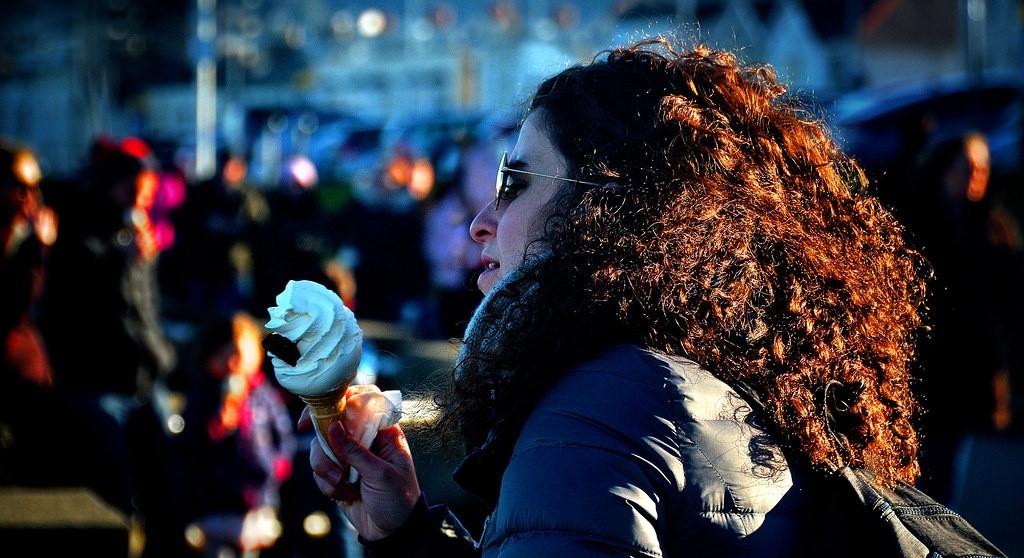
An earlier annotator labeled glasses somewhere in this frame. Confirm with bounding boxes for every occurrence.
[494,151,602,211]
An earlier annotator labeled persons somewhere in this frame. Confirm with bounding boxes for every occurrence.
[0,130,476,541]
[922,129,1024,542]
[297,33,937,558]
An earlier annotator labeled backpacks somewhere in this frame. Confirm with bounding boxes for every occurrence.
[728,376,1009,558]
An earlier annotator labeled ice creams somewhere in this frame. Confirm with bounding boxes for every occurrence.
[261,280,362,479]
